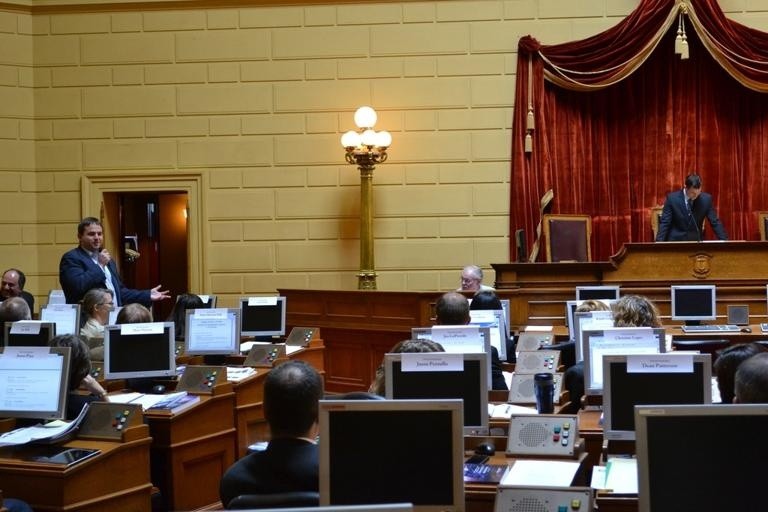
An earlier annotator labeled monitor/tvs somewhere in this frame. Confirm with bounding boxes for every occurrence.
[573,311,611,362]
[601,354,712,443]
[39,305,82,336]
[0,343,73,422]
[467,299,511,339]
[408,327,493,390]
[383,352,489,439]
[46,289,67,306]
[575,285,620,299]
[183,307,241,364]
[316,399,464,511]
[477,440,497,456]
[667,284,717,325]
[467,309,507,361]
[107,306,124,326]
[577,327,666,396]
[566,300,617,340]
[238,297,287,342]
[176,295,218,309]
[153,380,167,393]
[3,319,55,346]
[632,403,768,511]
[102,320,179,386]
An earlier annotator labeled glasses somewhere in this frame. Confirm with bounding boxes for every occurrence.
[97,300,114,306]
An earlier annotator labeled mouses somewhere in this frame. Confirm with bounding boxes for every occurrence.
[735,326,751,335]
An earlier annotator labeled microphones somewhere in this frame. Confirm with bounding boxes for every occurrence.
[684,196,703,241]
[97,246,111,268]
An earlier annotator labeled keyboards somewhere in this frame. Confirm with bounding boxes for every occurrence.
[680,323,740,334]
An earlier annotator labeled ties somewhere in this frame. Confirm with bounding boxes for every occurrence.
[687,198,693,215]
[93,253,100,264]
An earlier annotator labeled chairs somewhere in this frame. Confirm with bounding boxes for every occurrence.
[649,206,706,241]
[757,210,768,242]
[543,214,592,264]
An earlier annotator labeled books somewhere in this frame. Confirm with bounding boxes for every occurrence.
[464,464,510,484]
[224,367,258,383]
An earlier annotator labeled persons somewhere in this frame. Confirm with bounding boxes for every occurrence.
[612,293,661,327]
[575,298,607,313]
[653,171,727,241]
[0,268,205,431]
[453,264,494,300]
[434,289,507,390]
[367,338,447,400]
[712,341,761,404]
[470,291,516,365]
[732,352,768,404]
[217,360,326,509]
[57,216,173,327]
